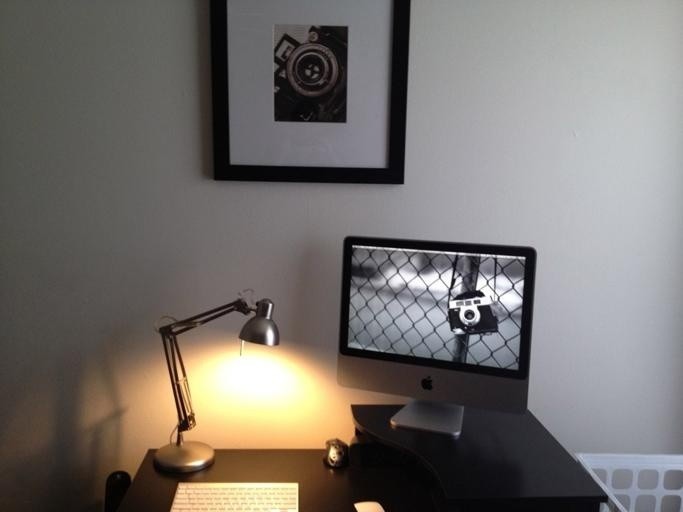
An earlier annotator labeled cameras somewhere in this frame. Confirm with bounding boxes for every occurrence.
[274,27,348,121]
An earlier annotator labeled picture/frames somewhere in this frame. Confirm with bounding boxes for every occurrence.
[210,0,412,183]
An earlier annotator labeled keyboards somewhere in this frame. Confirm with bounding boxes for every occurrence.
[169,481,300,512]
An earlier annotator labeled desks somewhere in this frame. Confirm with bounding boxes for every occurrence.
[122,402,605,512]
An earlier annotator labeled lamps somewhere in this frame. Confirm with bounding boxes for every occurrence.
[152,296,282,470]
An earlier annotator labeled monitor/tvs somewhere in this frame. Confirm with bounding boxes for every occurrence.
[448,291,498,335]
[337,236,537,438]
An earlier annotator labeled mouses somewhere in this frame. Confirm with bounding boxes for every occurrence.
[354,501,384,511]
